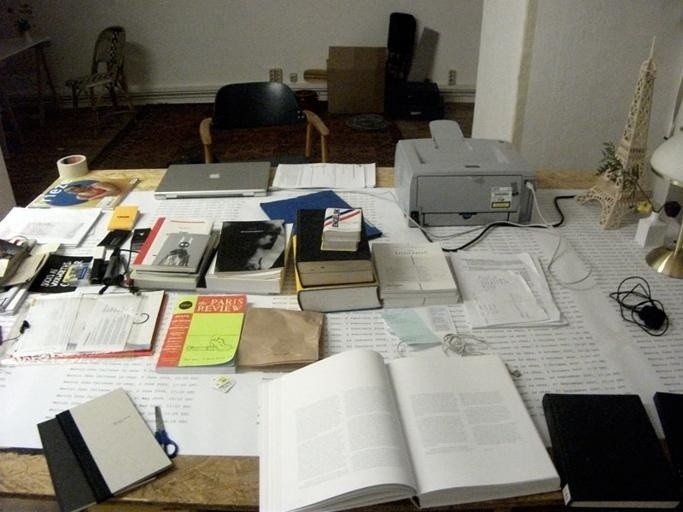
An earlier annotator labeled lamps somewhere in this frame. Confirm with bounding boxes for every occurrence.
[646,132,683,280]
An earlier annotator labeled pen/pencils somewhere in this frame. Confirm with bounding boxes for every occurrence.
[103,247,120,286]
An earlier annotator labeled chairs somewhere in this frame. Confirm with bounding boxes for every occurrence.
[68,26,135,126]
[197,78,330,164]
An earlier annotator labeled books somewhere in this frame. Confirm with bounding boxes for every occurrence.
[541,393,680,508]
[258,348,563,512]
[653,390,682,469]
[28,175,138,212]
[36,417,156,511]
[55,386,174,504]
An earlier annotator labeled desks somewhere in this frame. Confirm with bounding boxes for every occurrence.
[0,162,683,510]
[0,33,66,148]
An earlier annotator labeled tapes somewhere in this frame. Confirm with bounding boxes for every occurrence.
[56,155,89,180]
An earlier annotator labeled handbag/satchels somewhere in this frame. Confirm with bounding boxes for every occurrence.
[400,80,443,120]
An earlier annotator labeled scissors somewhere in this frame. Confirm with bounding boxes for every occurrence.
[155,407,178,459]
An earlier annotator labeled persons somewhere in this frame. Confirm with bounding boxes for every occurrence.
[61,182,123,202]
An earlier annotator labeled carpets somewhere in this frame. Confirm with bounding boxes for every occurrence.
[89,97,407,203]
